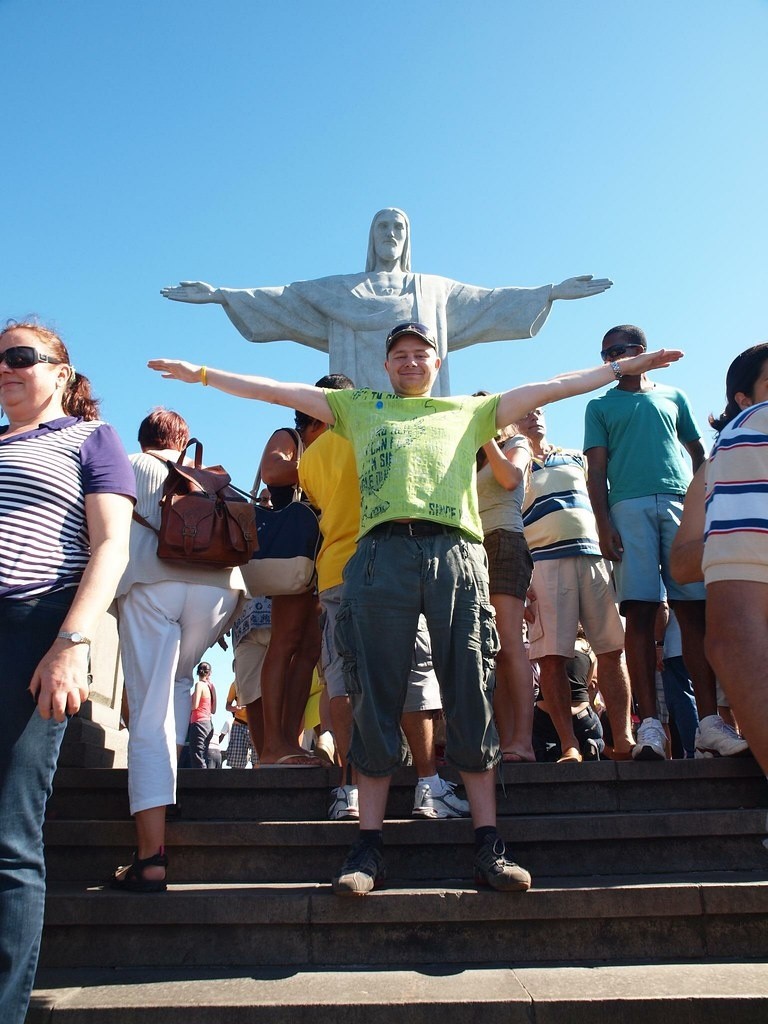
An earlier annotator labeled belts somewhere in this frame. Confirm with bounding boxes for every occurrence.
[379,521,455,538]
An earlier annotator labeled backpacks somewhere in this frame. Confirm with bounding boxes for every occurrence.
[132,437,259,568]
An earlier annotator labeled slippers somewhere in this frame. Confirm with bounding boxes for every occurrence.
[502,751,531,763]
[259,753,323,767]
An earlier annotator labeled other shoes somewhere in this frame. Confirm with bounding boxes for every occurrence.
[611,749,631,760]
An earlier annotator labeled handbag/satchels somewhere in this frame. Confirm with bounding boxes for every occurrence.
[238,422,322,597]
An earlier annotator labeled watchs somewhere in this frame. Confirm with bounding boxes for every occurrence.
[58,631,91,644]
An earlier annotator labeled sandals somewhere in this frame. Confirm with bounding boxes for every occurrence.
[106,848,167,893]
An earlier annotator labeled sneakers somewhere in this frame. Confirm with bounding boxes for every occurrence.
[332,836,384,896]
[693,714,749,759]
[411,779,470,819]
[327,784,360,820]
[632,716,668,759]
[474,831,531,891]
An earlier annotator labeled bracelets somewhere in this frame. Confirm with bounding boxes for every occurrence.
[610,361,622,380]
[202,365,207,386]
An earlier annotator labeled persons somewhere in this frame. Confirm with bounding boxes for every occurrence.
[148,320,684,897]
[223,325,748,819]
[669,342,768,779]
[107,409,251,895]
[0,321,137,1024]
[160,208,613,396]
[191,662,216,769]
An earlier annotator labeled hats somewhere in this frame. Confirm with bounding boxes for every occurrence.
[385,322,438,358]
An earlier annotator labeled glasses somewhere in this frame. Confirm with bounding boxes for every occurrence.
[0,346,62,369]
[601,343,647,361]
